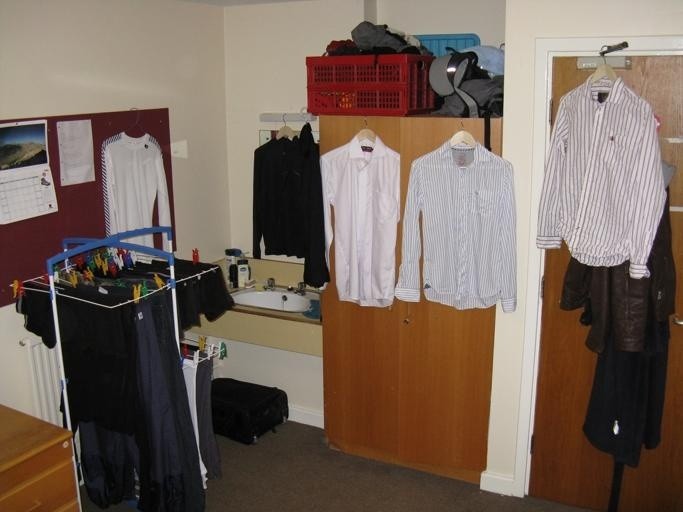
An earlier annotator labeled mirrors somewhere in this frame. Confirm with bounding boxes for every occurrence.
[259,130,319,146]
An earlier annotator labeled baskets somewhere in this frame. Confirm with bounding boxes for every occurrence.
[305,54,441,117]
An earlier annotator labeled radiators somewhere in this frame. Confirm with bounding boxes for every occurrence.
[20,335,64,429]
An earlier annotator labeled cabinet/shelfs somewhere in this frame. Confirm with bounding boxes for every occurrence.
[319,115,502,486]
[0,405,80,512]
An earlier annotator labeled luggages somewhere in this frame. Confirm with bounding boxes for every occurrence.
[211,377,289,446]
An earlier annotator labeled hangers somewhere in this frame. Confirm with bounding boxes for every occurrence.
[449,116,476,147]
[299,106,309,124]
[125,108,145,134]
[357,114,376,142]
[589,44,618,86]
[276,113,296,140]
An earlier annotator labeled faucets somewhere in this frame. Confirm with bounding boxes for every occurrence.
[263,278,275,291]
[294,282,306,296]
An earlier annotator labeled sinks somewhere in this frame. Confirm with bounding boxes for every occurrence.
[228,286,320,312]
